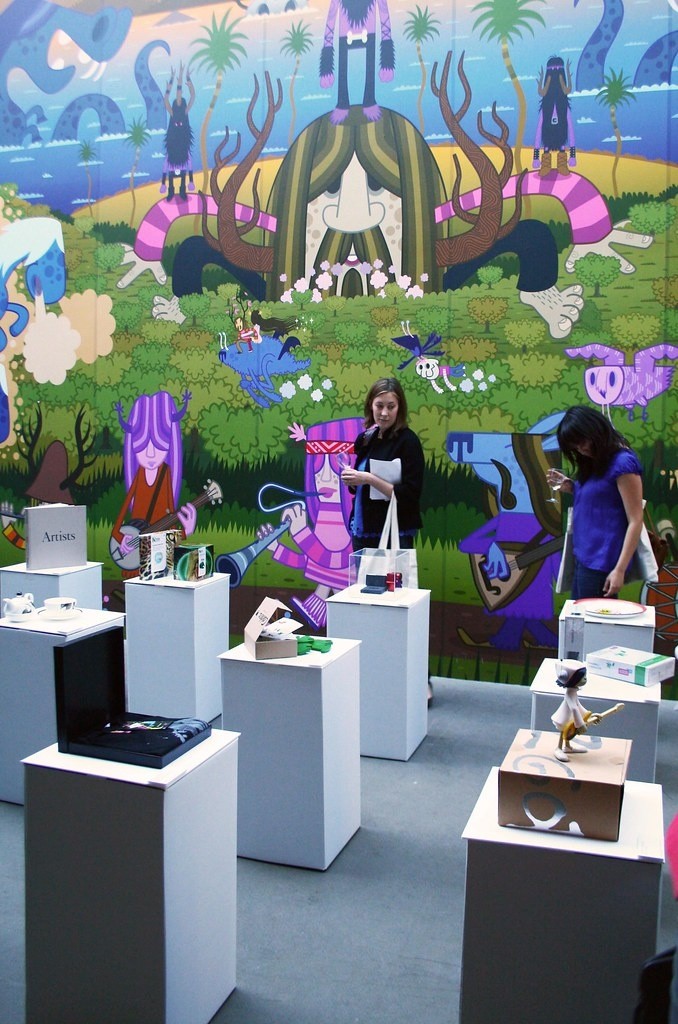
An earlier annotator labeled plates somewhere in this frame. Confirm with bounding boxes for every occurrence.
[572,597,647,619]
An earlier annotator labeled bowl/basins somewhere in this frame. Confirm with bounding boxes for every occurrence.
[44,597,77,617]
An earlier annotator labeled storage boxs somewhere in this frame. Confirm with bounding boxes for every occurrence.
[23,505,88,571]
[347,547,418,602]
[586,644,676,686]
[243,595,298,660]
[53,626,213,771]
[138,529,215,581]
[497,728,634,844]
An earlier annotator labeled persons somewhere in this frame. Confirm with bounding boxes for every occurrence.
[544,405,644,601]
[551,659,603,762]
[340,377,434,709]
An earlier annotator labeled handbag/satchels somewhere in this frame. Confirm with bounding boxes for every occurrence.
[356,491,417,591]
[624,500,659,584]
[555,506,574,594]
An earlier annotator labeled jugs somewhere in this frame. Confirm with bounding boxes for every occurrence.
[2,591,37,622]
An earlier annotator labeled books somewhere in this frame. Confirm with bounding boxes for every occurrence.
[23,502,88,571]
